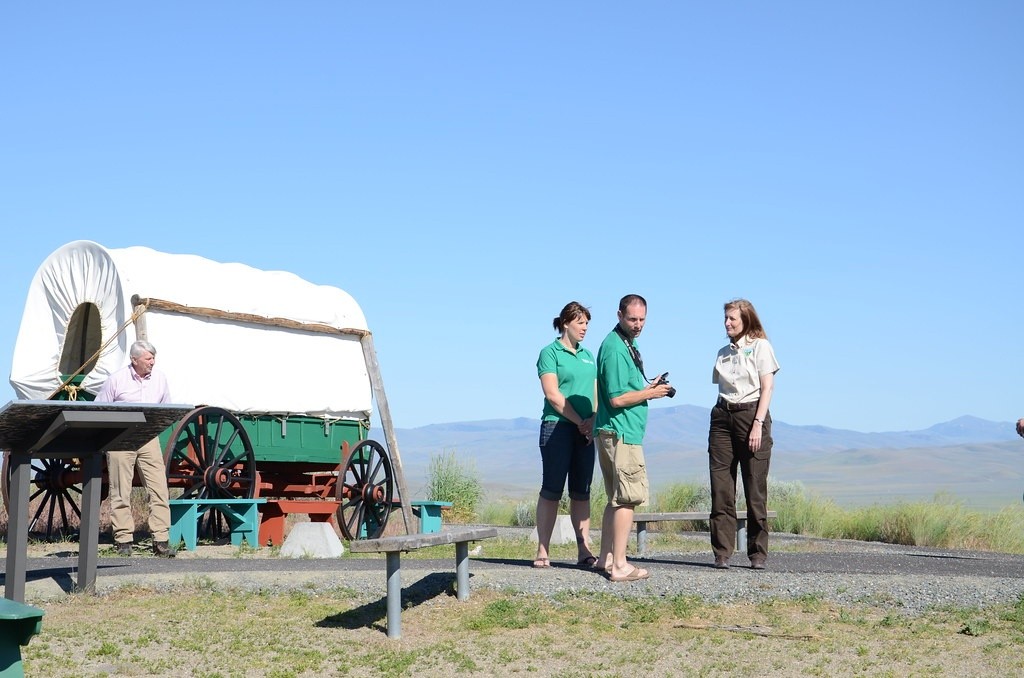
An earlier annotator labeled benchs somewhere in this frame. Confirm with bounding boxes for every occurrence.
[357,500,453,540]
[257,500,345,547]
[351,527,499,640]
[166,498,267,550]
[631,510,778,555]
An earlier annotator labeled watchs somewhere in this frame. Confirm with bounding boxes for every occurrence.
[754,419,764,423]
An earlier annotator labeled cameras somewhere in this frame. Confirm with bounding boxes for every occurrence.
[656,372,676,398]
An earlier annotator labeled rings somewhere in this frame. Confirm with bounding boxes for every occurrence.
[586,430,590,434]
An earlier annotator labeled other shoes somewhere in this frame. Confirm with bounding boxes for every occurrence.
[751,557,766,569]
[714,557,732,567]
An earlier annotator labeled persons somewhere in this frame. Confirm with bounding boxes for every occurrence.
[707,298,779,569]
[532,300,599,568]
[594,293,671,583]
[94,340,175,558]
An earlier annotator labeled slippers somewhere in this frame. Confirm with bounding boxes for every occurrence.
[608,565,649,581]
[534,558,550,568]
[577,556,598,567]
[592,565,612,572]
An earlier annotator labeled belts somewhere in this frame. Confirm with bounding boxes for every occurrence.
[718,397,760,410]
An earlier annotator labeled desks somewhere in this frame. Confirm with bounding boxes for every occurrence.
[0,400,197,607]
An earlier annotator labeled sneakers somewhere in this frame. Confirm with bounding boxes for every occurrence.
[118,543,133,555]
[153,541,175,557]
